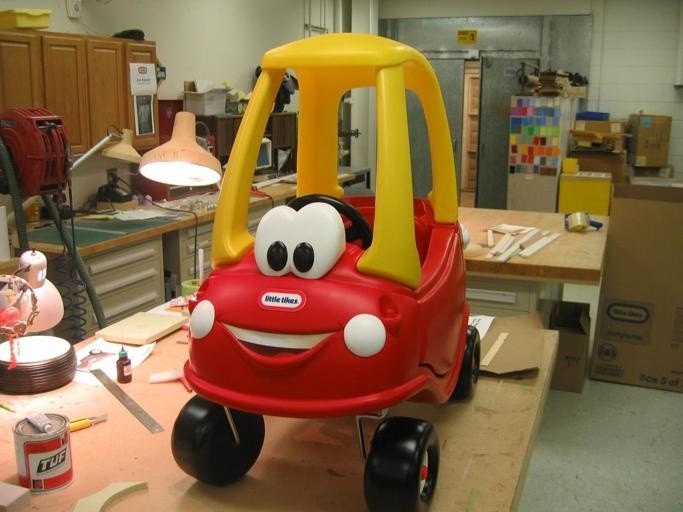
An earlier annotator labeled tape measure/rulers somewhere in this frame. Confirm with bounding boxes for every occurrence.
[90,369,165,435]
[481,332,510,366]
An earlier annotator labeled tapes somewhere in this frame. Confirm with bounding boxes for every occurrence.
[568,212,587,231]
[180,279,199,296]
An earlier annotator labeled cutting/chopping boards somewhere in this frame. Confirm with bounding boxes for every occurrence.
[94,312,187,345]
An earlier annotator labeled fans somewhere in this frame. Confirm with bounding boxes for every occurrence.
[66,0,110,19]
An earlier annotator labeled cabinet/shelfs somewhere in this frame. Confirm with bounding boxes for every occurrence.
[195,115,297,155]
[0,27,160,157]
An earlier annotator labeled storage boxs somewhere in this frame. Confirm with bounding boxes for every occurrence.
[547,304,591,394]
[568,113,683,393]
[179,79,233,118]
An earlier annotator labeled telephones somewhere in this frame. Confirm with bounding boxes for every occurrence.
[97,184,132,202]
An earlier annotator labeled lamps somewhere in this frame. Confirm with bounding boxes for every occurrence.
[138,111,224,187]
[0,247,66,335]
[69,124,142,174]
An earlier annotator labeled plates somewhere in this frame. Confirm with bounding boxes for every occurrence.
[1,335,77,394]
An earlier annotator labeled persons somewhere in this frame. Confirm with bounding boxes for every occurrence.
[138,96,151,134]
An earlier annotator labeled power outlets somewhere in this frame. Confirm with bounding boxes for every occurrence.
[106,167,118,186]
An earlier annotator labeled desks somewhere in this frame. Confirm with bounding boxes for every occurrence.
[0,293,560,512]
[10,171,359,343]
[440,207,609,318]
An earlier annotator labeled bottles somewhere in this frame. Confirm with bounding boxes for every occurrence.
[117,346,132,384]
[133,194,152,207]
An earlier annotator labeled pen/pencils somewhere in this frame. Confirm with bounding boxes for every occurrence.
[173,367,192,392]
[177,340,188,344]
[0,404,17,414]
